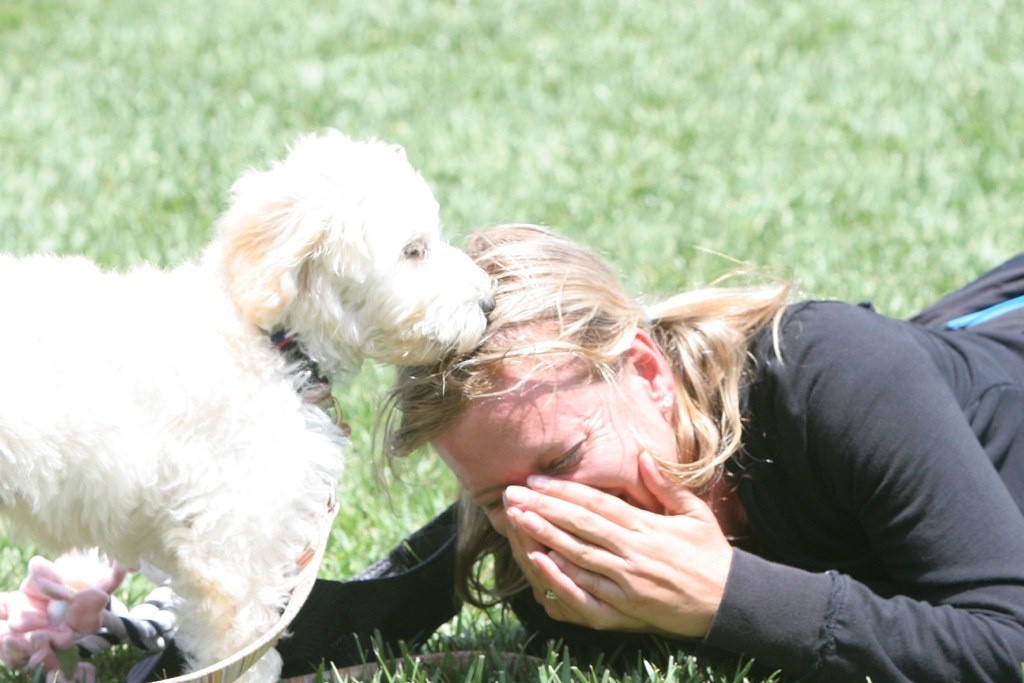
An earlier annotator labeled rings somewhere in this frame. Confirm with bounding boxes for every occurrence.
[544,590,558,600]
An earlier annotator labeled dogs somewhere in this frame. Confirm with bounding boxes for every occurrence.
[0,128,497,675]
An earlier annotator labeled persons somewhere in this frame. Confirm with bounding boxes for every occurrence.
[0,222,1024,683]
